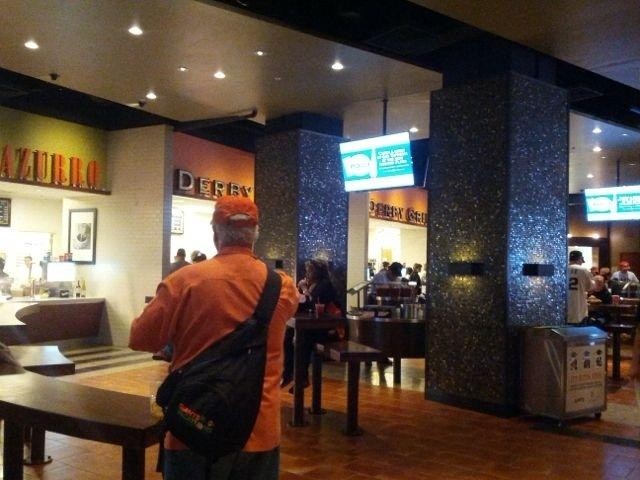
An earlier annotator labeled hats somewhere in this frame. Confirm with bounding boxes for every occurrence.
[212,193,260,226]
[569,251,585,263]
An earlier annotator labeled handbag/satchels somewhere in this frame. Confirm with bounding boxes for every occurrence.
[327,304,345,341]
[156,317,267,461]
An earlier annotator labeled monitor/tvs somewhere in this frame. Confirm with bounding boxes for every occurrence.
[583,185,640,223]
[411,137,429,189]
[338,130,415,193]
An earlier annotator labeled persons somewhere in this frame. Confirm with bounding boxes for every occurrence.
[0,253,12,296]
[566,251,640,327]
[171,248,192,273]
[191,250,206,263]
[128,196,301,480]
[366,261,422,304]
[280,259,331,394]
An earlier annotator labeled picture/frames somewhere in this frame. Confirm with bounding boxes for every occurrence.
[67,208,97,264]
[0,198,11,227]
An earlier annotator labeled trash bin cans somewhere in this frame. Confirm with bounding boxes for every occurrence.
[519,323,611,426]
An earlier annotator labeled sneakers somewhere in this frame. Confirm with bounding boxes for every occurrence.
[288,380,311,394]
[279,377,293,389]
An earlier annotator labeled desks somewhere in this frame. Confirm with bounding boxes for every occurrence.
[286,312,344,427]
[585,297,640,381]
[346,314,425,384]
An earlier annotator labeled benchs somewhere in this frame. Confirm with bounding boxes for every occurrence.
[308,341,383,436]
[0,361,165,480]
[8,345,74,465]
